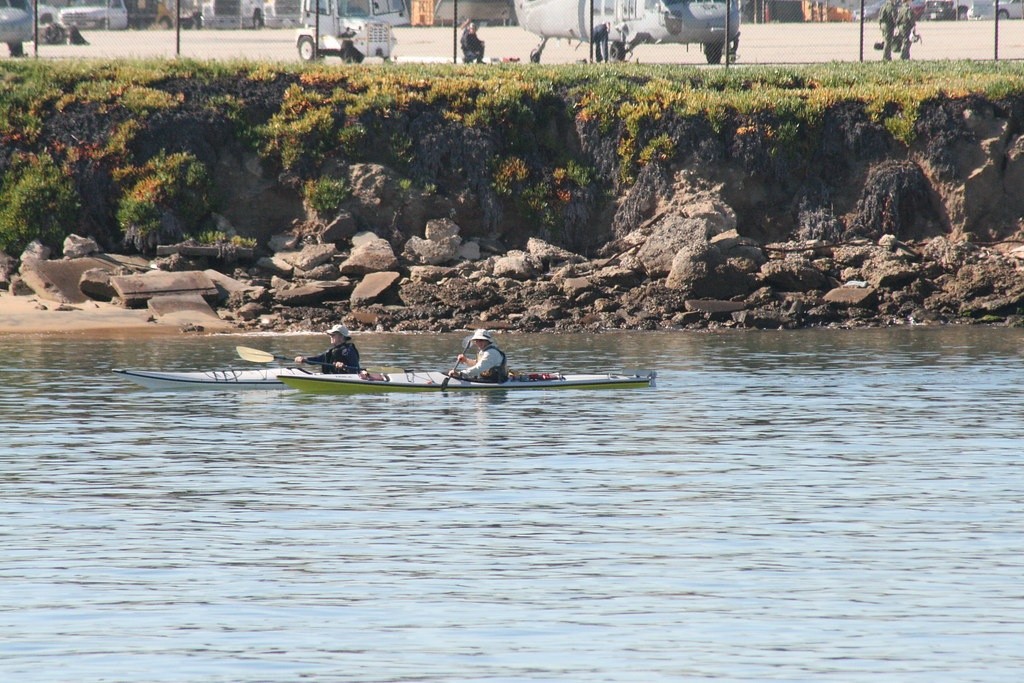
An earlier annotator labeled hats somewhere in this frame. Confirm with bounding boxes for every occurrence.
[471,328,493,343]
[327,324,351,340]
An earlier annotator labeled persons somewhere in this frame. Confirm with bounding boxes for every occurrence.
[592,22,610,63]
[461,23,486,64]
[295,325,360,375]
[461,13,471,49]
[878,0,916,62]
[39,21,91,46]
[448,329,508,384]
[249,0,267,30]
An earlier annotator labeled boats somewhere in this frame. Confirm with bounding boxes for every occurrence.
[110,366,416,393]
[275,362,658,396]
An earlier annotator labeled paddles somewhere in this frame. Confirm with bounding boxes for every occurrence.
[235,345,367,372]
[441,335,476,392]
